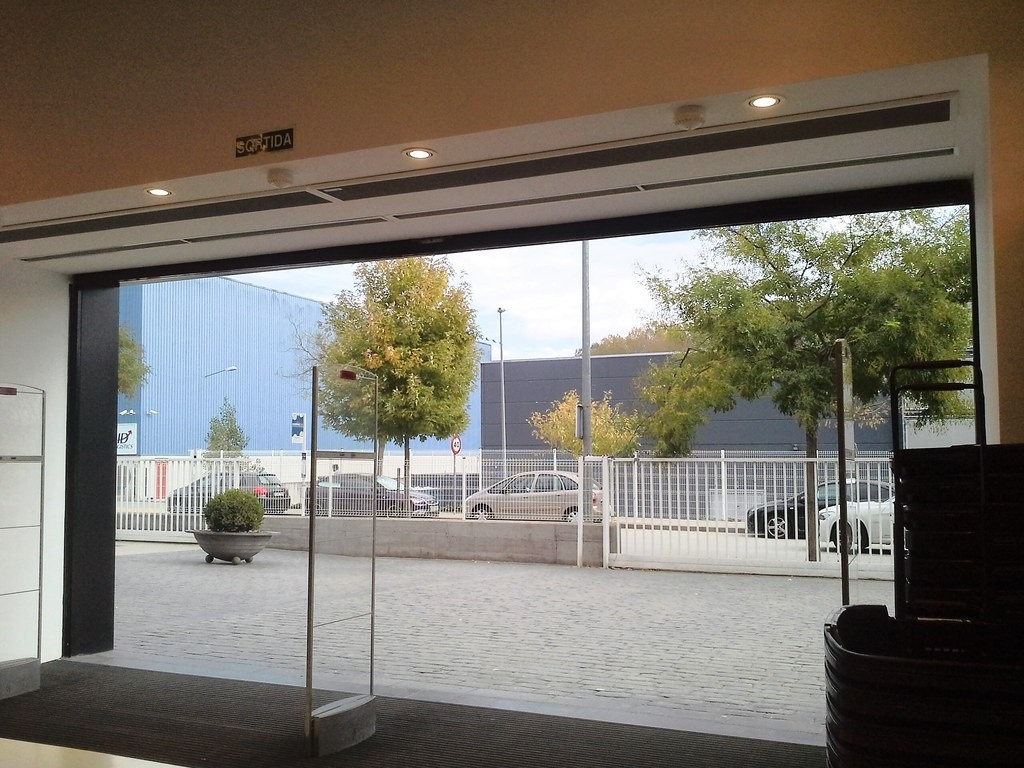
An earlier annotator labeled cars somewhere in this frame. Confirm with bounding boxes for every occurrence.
[819,496,895,556]
[317,472,440,518]
[168,470,291,513]
[464,472,605,523]
[748,479,896,539]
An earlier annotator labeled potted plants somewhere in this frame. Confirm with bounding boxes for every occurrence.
[185,483,274,568]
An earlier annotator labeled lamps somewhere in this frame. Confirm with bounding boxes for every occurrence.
[204,364,237,377]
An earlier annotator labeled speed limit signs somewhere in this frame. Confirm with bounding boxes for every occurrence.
[451,435,462,454]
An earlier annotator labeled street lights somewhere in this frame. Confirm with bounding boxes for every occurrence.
[497,306,510,479]
